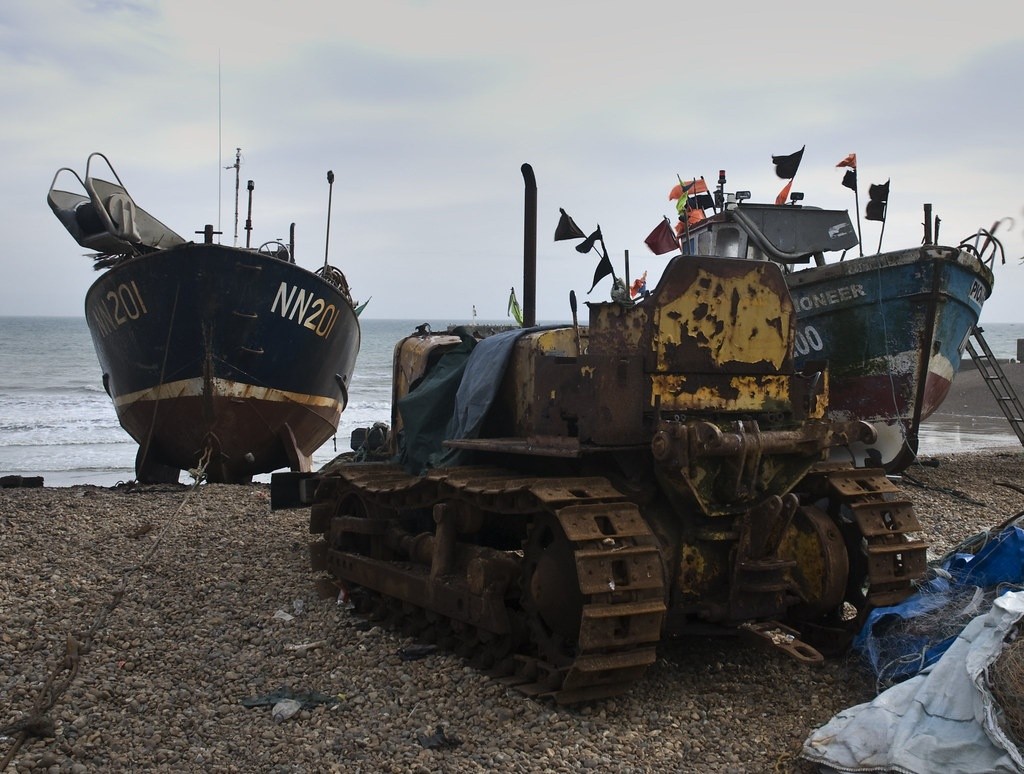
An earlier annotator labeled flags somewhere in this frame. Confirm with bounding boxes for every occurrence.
[506,143,890,329]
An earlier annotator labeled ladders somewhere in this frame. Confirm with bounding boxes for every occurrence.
[965,323,1024,450]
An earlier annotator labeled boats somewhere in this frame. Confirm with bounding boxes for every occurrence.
[84,147,364,484]
[634,167,999,476]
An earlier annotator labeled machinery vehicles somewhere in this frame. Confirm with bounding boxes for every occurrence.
[269,158,931,701]
